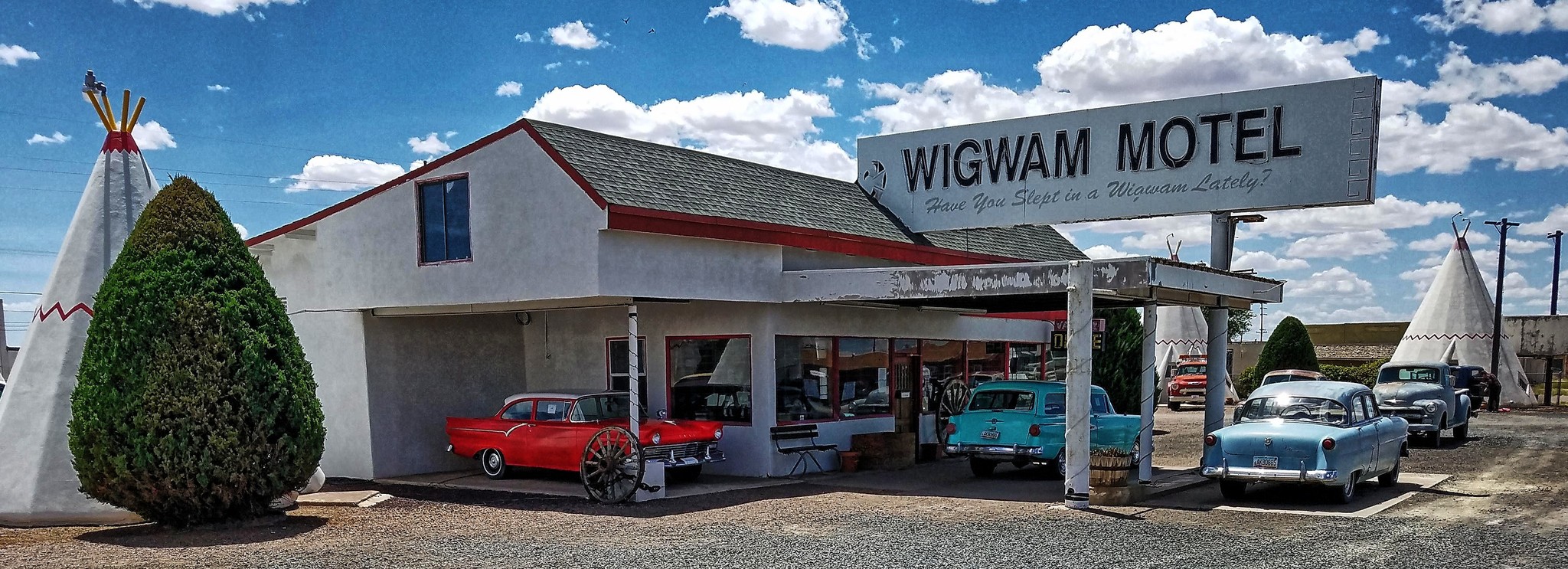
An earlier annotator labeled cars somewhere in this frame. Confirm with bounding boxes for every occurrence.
[943,379,1141,479]
[444,388,725,482]
[1232,369,1326,425]
[1449,365,1495,398]
[671,369,1005,424]
[1200,381,1410,506]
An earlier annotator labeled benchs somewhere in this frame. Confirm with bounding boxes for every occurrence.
[769,424,842,481]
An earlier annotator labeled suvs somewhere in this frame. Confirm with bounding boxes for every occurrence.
[1168,354,1207,412]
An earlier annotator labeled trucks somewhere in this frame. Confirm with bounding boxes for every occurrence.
[1372,361,1472,449]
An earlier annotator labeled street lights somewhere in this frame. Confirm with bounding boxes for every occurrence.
[1484,218,1520,409]
[1543,229,1565,405]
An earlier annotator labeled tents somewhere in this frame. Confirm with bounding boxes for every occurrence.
[1134,234,1241,406]
[1389,211,1540,408]
[0,88,327,530]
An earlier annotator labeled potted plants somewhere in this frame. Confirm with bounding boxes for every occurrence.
[1089,447,1132,487]
[839,450,862,473]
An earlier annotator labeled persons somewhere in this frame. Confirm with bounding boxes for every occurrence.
[1479,370,1502,413]
